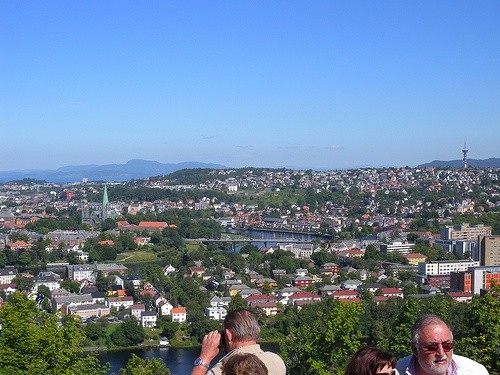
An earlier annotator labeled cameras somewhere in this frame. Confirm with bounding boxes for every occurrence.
[218,330,226,345]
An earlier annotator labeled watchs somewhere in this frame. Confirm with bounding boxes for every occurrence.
[193,357,210,369]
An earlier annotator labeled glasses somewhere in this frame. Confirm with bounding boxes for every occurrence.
[427,342,454,352]
[376,370,396,375]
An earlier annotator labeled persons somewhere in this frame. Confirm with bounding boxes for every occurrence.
[394,315,489,375]
[190,308,286,375]
[221,353,268,375]
[344,345,396,375]
